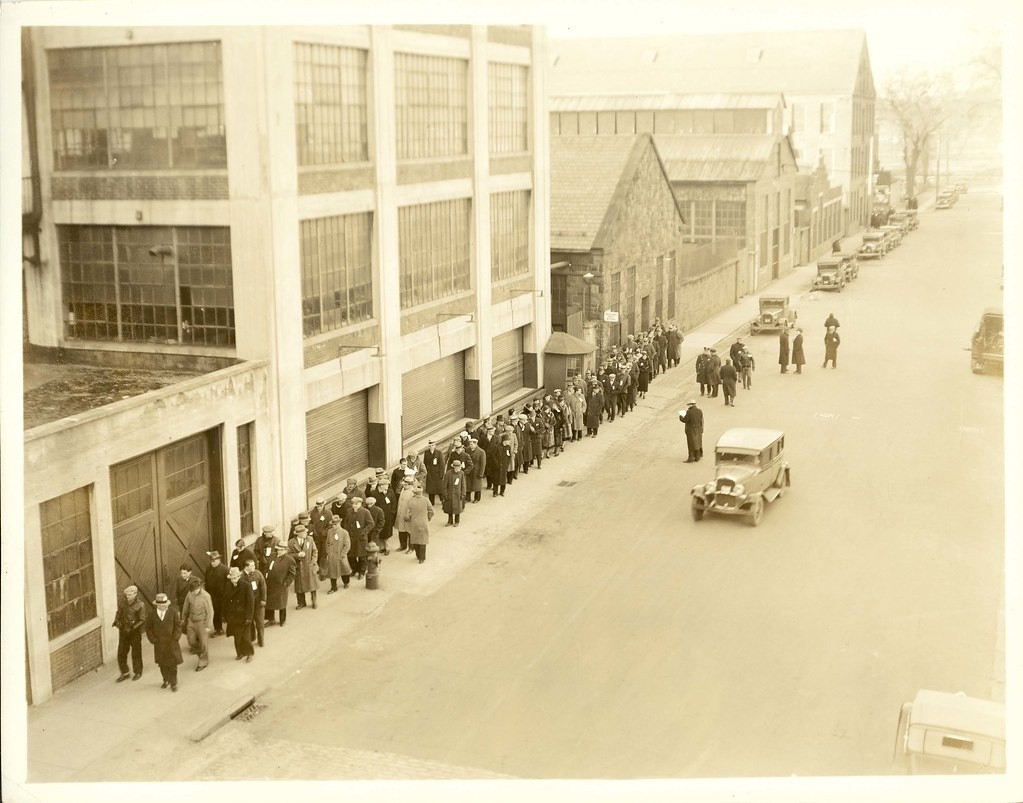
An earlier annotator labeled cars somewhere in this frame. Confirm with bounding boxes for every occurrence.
[936,184,967,208]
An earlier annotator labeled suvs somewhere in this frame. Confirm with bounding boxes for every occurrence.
[879,209,921,249]
[833,252,859,281]
[750,295,798,335]
[858,232,888,260]
[688,429,794,526]
[813,257,847,292]
[972,309,1004,374]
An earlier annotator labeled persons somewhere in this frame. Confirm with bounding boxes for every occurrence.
[779,328,803,374]
[822,313,840,370]
[679,399,704,463]
[113,319,684,692]
[696,337,755,407]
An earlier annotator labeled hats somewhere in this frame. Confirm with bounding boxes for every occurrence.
[687,400,697,405]
[123,551,242,605]
[235,498,343,549]
[428,413,514,467]
[336,450,423,505]
[704,312,841,365]
[599,315,679,380]
[508,369,600,420]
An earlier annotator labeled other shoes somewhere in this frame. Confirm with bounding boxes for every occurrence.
[523,429,596,475]
[699,379,752,407]
[599,391,646,424]
[104,627,263,691]
[440,473,517,528]
[378,543,425,565]
[694,457,700,462]
[683,458,694,463]
[262,561,366,627]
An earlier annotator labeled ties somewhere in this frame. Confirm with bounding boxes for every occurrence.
[300,539,302,547]
